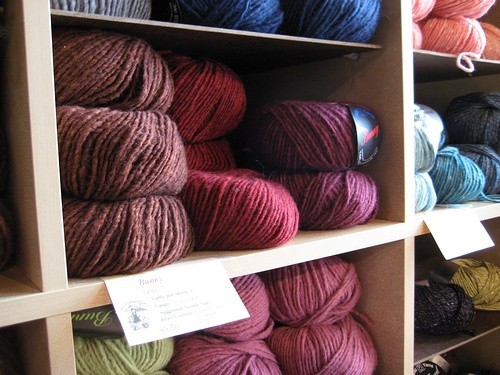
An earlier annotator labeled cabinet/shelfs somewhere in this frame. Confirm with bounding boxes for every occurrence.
[0,1,500,375]
